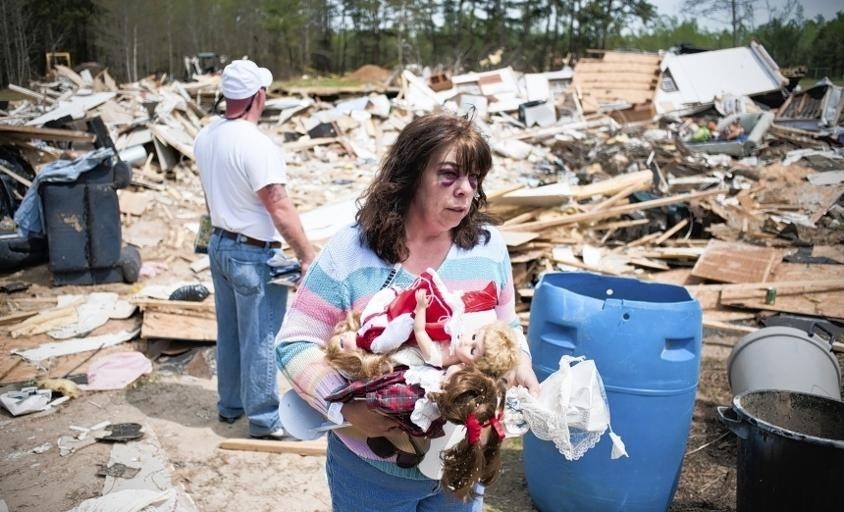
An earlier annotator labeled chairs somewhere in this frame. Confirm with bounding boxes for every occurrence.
[39,154,141,286]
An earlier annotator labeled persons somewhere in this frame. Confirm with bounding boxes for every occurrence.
[195,60,317,441]
[325,268,520,504]
[325,268,519,504]
[274,113,540,512]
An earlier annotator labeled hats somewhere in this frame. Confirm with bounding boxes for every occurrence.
[222,60,273,100]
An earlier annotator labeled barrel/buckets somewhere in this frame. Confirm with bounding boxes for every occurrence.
[716,388,844,511]
[522,269,701,512]
[723,328,840,397]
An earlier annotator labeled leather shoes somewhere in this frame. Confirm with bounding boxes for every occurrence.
[214,227,281,249]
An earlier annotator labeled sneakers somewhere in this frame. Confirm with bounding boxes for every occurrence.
[250,427,302,441]
[219,412,244,423]
